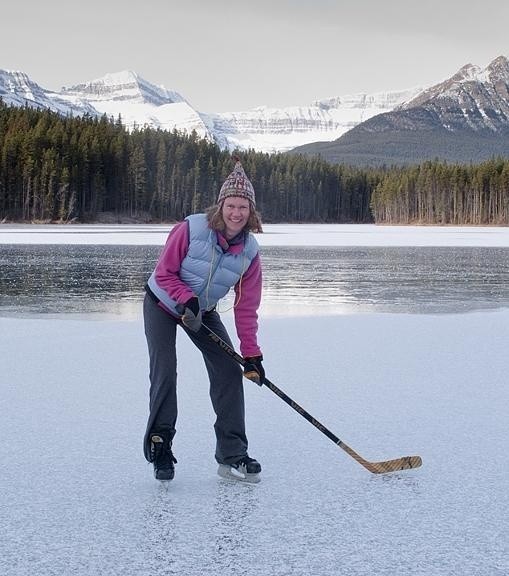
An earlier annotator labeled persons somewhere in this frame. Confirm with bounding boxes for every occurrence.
[142,161,267,481]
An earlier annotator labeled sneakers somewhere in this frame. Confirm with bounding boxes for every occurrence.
[151,431,176,481]
[214,452,262,475]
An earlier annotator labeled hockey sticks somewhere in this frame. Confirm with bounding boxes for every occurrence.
[175,302,422,473]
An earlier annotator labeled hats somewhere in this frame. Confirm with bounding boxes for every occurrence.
[217,156,256,205]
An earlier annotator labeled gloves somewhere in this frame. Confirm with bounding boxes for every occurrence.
[180,296,203,334]
[243,356,266,388]
